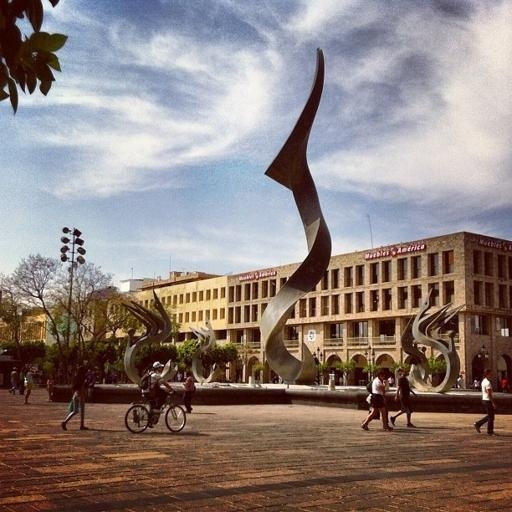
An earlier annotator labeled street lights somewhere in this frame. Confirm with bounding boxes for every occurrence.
[477,343,492,361]
[313,346,324,386]
[57,224,86,385]
[364,345,376,382]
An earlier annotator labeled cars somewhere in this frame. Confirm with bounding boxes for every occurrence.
[28,373,41,384]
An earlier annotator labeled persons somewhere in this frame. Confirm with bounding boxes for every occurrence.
[141,361,175,424]
[61,367,88,431]
[9,367,56,404]
[183,371,195,413]
[361,368,509,436]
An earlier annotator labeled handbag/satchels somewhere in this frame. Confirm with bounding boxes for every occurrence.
[367,381,372,393]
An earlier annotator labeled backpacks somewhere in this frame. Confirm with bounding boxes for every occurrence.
[139,372,157,390]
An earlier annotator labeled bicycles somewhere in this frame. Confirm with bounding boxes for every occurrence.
[124,389,187,435]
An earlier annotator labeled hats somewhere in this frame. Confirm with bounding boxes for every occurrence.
[153,362,164,368]
